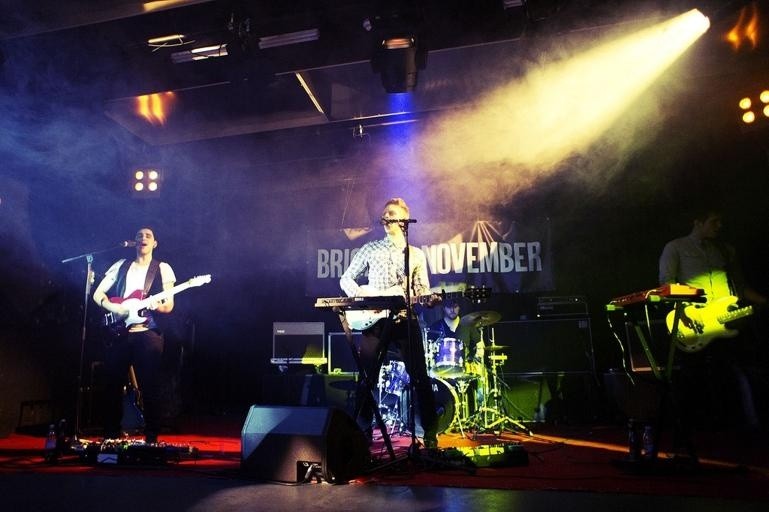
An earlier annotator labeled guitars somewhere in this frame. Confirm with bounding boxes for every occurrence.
[96,274,212,342]
[345,285,492,331]
[666,295,769,352]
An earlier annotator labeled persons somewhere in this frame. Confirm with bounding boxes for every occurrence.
[427,300,485,434]
[340,198,443,450]
[644,204,767,473]
[93,226,177,443]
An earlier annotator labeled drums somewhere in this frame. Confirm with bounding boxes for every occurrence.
[397,377,460,438]
[385,359,410,396]
[433,337,466,374]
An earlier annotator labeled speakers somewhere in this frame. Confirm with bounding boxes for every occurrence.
[240,404,366,483]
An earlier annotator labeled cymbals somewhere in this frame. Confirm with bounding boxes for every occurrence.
[458,310,503,328]
[477,343,512,352]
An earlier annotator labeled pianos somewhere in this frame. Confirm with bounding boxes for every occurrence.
[610,284,705,310]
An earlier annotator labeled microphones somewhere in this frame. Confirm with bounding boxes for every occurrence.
[123,240,139,248]
[380,216,417,225]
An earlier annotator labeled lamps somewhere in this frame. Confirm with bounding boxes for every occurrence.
[367,1,434,97]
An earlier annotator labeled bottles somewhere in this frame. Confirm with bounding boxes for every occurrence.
[627,416,654,465]
[45,423,57,459]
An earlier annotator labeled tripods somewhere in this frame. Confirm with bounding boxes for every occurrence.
[373,323,533,441]
[362,238,475,477]
[61,265,96,455]
